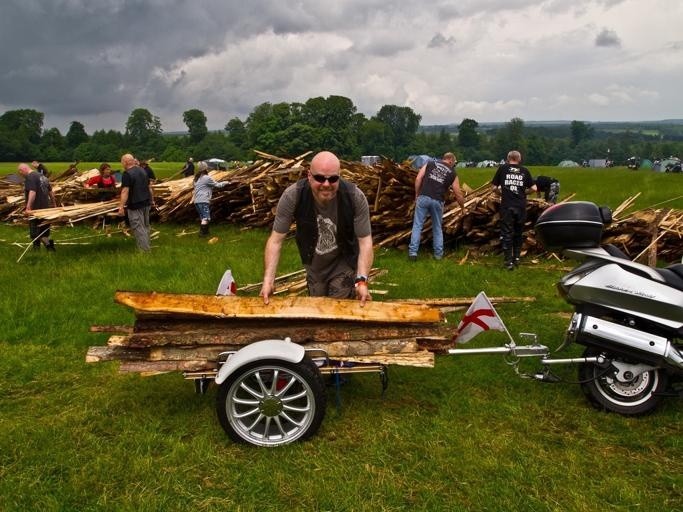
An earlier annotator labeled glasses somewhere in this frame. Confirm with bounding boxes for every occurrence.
[309,169,340,184]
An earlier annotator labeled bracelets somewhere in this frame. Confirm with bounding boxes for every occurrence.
[353,274,368,288]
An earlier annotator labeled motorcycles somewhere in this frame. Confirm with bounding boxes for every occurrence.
[530,197,682,418]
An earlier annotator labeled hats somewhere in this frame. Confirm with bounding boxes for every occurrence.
[196,161,207,171]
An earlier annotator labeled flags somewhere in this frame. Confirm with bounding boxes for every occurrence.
[452,291,505,344]
[216,269,237,296]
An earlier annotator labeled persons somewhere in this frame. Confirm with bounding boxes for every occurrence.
[119,154,155,251]
[408,152,467,262]
[18,163,56,252]
[259,151,374,307]
[530,176,560,203]
[180,157,194,178]
[81,163,130,237]
[32,160,48,175]
[490,151,538,270]
[189,161,233,237]
[135,156,156,181]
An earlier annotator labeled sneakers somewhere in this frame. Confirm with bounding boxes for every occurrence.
[408,255,418,263]
[502,258,520,271]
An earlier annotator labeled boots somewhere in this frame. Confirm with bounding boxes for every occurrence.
[32,240,56,254]
[198,224,210,237]
[100,220,130,239]
